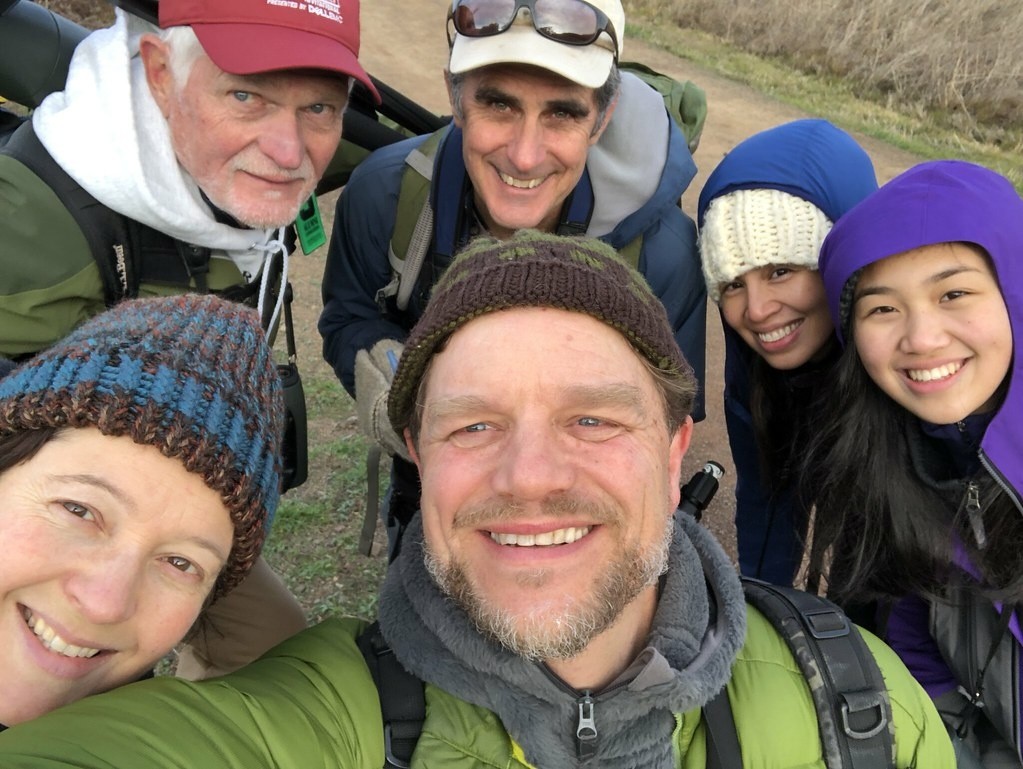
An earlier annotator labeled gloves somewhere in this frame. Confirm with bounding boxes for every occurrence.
[355,336,416,467]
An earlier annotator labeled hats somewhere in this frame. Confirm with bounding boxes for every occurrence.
[695,186,838,304]
[449,0,626,89]
[388,228,700,446]
[158,0,383,107]
[0,292,285,607]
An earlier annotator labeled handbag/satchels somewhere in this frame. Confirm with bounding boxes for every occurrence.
[268,364,308,493]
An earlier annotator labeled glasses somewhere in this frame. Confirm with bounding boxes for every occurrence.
[445,0,618,68]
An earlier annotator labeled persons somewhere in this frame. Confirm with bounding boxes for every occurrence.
[316,0,708,565]
[0,291,309,734]
[692,113,882,595]
[0,0,384,382]
[813,156,1023,768]
[0,226,957,769]
